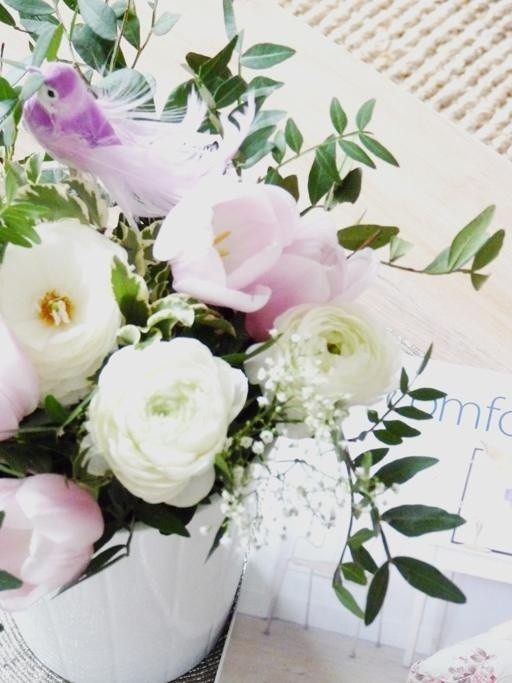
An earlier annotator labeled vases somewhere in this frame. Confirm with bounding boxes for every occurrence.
[0,482,246,682]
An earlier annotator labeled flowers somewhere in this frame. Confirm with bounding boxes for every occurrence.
[0,0,508,628]
